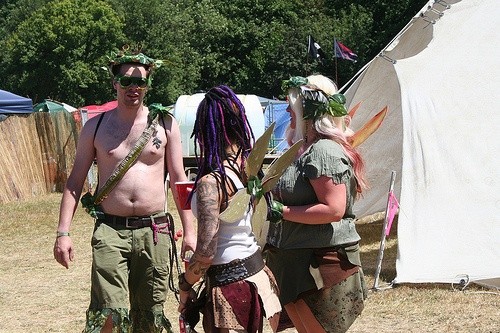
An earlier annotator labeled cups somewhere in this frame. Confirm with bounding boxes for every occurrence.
[183,259,190,272]
[175,182,195,210]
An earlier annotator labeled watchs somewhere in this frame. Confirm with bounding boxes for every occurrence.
[56,231,70,238]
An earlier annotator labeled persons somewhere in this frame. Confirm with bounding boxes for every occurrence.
[250,72,388,333]
[53,47,198,333]
[177,85,304,333]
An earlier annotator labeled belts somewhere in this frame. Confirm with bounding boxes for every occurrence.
[101,214,168,230]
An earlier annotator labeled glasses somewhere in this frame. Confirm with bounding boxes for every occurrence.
[114,77,150,89]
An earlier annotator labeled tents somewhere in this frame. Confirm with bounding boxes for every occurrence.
[167,0,500,293]
[0,89,118,122]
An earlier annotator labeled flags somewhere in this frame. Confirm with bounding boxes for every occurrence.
[309,34,327,65]
[334,39,359,64]
